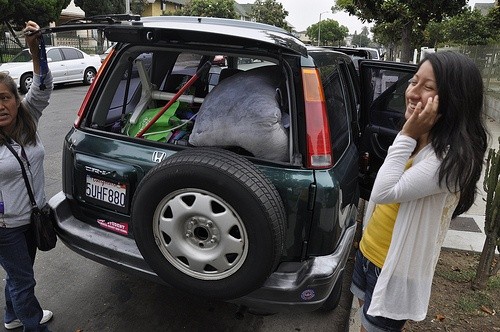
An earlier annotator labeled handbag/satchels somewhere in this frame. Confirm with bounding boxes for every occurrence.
[123,101,190,143]
[30,202,58,251]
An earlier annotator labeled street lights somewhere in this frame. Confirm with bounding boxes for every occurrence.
[317,12,334,47]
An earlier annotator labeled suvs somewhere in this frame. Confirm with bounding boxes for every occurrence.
[12,18,423,315]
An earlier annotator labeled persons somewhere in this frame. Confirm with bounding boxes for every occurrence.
[0,20,55,332]
[348,49,494,332]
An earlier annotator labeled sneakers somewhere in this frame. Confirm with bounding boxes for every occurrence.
[4,309,53,329]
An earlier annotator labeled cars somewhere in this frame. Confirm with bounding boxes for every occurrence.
[0,46,102,94]
[101,43,153,81]
[238,57,264,62]
[317,45,381,77]
[212,54,230,66]
